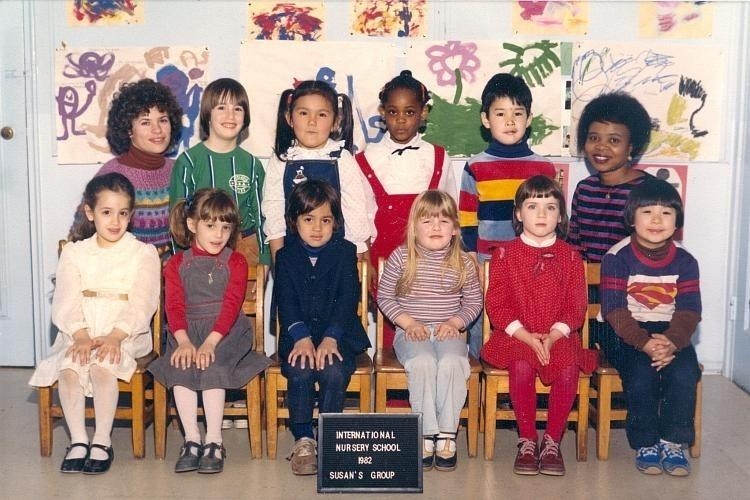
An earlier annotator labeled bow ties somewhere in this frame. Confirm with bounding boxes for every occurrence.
[392,145,420,156]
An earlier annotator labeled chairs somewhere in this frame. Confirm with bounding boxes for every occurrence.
[151,261,266,460]
[374,252,482,459]
[35,238,165,460]
[581,260,706,460]
[482,259,596,462]
[266,261,374,459]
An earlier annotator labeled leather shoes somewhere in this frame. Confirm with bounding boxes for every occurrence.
[59,441,90,474]
[82,444,114,475]
[175,440,204,473]
[198,443,226,473]
[434,434,458,472]
[422,437,434,472]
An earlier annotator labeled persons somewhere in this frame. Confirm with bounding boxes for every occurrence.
[261,81,375,339]
[479,173,602,476]
[460,73,558,428]
[375,191,483,470]
[28,172,164,475]
[142,187,273,472]
[169,77,269,430]
[68,81,177,258]
[596,179,705,476]
[354,69,460,408]
[565,90,660,303]
[273,178,369,478]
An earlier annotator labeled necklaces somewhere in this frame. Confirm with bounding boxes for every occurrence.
[602,175,621,199]
[191,251,220,286]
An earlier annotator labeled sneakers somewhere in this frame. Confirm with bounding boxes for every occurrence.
[291,436,318,475]
[634,444,663,475]
[660,441,689,476]
[540,440,566,476]
[222,404,249,429]
[513,440,539,475]
